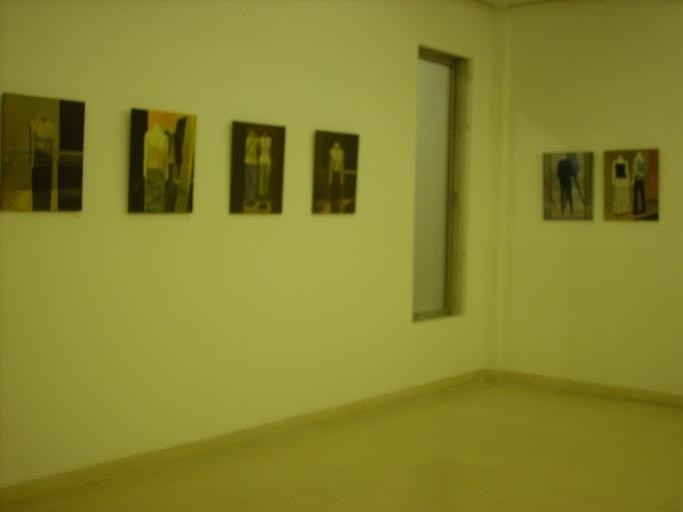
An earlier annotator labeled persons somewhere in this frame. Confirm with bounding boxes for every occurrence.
[327,141,345,213]
[244,128,272,209]
[28,111,58,212]
[612,151,647,216]
[142,122,171,213]
[556,153,579,215]
